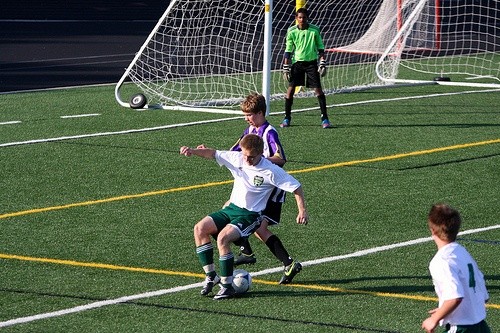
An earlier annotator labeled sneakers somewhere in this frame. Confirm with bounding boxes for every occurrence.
[279,259,303,284]
[321,118,330,128]
[201,272,221,295]
[213,283,237,299]
[279,117,290,128]
[233,251,256,265]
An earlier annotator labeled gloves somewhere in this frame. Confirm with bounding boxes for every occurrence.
[317,59,327,76]
[282,63,291,81]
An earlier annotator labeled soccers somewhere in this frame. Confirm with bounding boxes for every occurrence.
[231,268,251,297]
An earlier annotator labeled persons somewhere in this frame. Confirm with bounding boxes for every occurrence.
[421,204,493,332]
[180,133,309,300]
[278,7,332,130]
[198,92,303,285]
